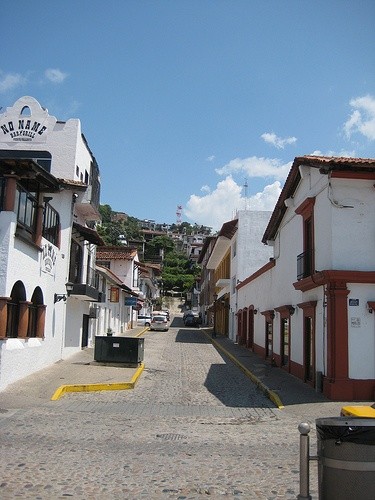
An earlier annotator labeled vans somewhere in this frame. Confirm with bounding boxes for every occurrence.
[137,314,151,326]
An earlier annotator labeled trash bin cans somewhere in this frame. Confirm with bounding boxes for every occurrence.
[298,416,374,500]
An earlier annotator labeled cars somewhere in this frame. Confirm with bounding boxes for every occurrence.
[183,311,196,326]
[153,309,169,320]
[150,316,168,331]
